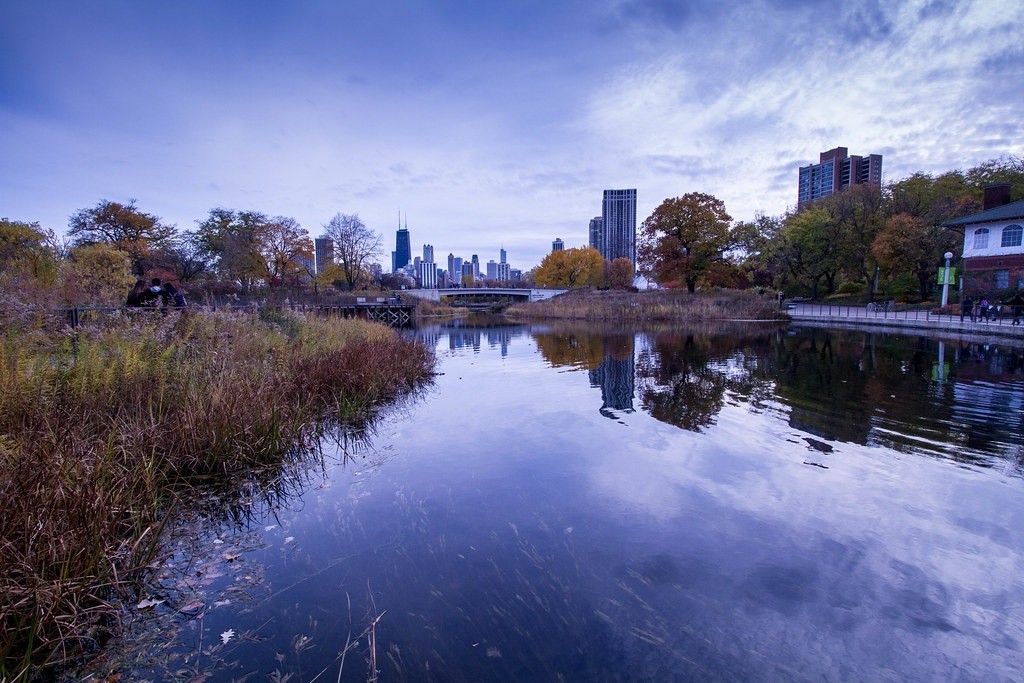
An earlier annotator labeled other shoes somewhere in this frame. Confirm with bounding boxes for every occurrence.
[979,320,982,322]
[1018,322,1021,325]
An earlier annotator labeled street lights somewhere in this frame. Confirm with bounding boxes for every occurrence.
[941,251,953,309]
[939,340,947,394]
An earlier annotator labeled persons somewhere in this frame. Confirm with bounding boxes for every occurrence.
[597,283,609,291]
[960,294,1024,325]
[406,282,546,290]
[778,289,784,308]
[122,278,187,319]
[393,292,401,304]
[759,288,764,297]
